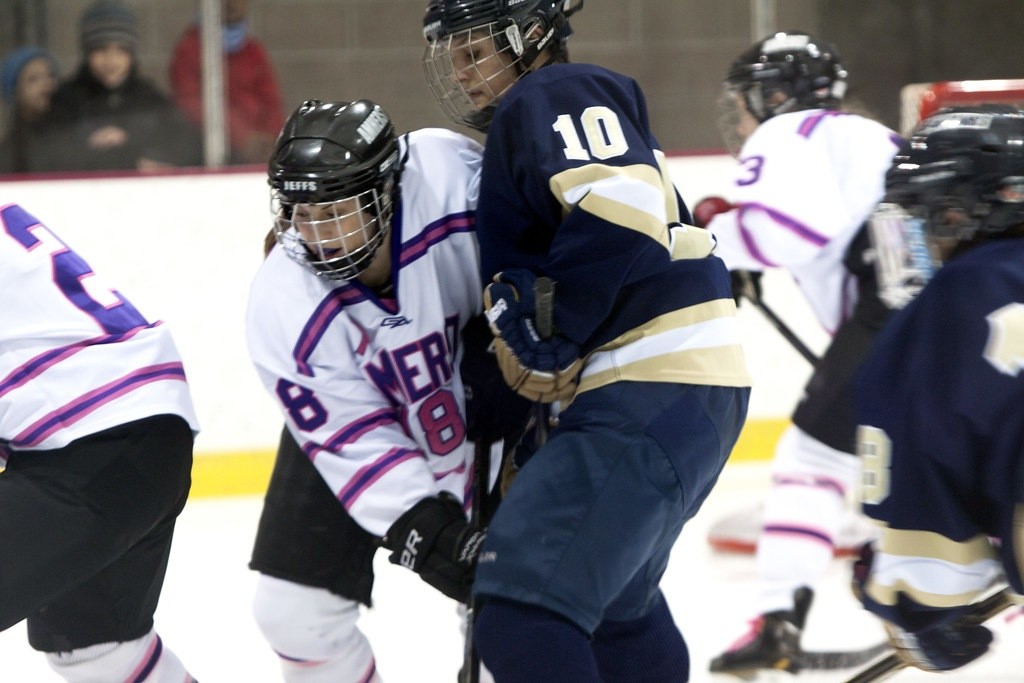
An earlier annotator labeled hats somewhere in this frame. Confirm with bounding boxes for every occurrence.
[78,0,139,54]
[0,48,53,105]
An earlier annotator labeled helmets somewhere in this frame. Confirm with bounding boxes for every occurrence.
[881,105,1024,235]
[268,97,403,282]
[716,30,851,159]
[422,0,570,120]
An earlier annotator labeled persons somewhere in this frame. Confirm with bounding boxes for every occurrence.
[33,1,243,171]
[0,47,67,173]
[849,103,1024,672]
[0,198,204,683]
[247,98,526,683]
[697,30,909,677]
[171,0,284,166]
[420,0,752,683]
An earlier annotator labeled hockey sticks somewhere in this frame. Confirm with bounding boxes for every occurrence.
[687,192,822,378]
[841,583,1023,683]
[460,314,492,683]
[515,273,565,475]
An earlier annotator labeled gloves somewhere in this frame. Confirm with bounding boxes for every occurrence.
[385,491,486,612]
[462,269,581,447]
[708,586,817,678]
